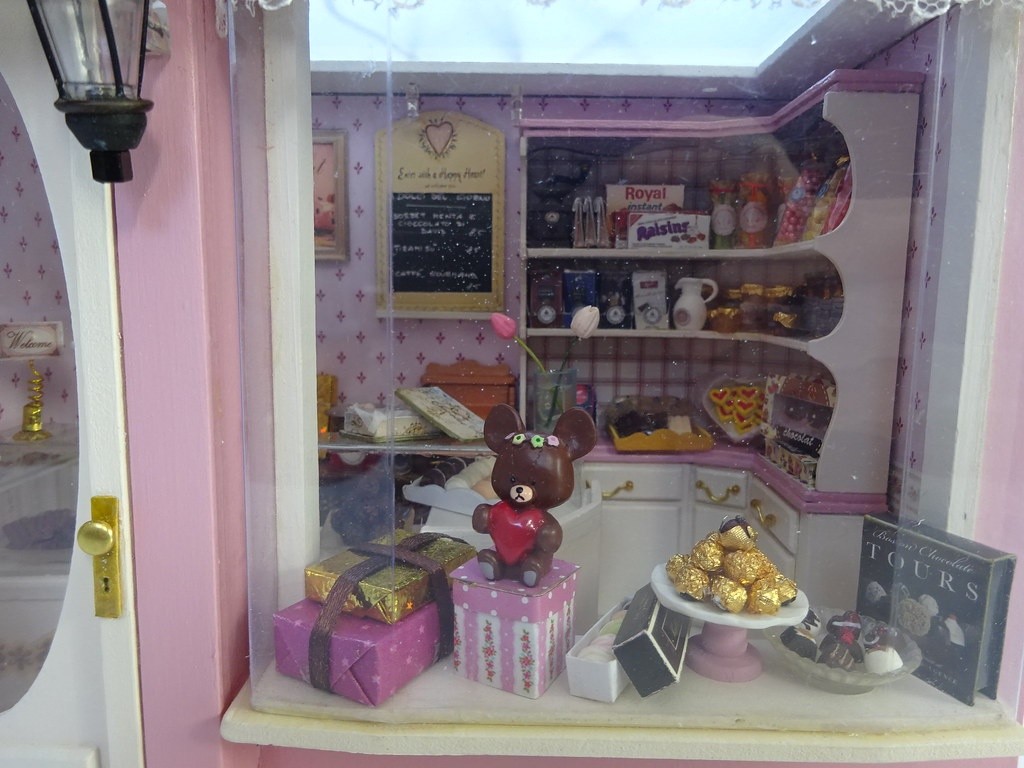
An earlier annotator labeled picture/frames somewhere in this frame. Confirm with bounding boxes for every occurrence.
[313,129,350,263]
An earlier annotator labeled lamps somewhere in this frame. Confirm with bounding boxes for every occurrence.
[27,0,167,183]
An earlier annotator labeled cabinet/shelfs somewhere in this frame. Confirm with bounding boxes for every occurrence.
[698,464,863,622]
[519,66,926,517]
[444,458,691,620]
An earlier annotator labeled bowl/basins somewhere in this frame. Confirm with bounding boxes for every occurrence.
[763,607,923,695]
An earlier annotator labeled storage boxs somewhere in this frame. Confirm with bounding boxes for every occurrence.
[337,387,485,442]
[450,545,586,698]
[273,594,452,707]
[606,185,685,237]
[304,529,474,629]
[615,203,721,249]
[564,597,630,703]
[765,376,834,487]
[612,578,692,705]
[532,266,671,332]
[856,514,1018,709]
[308,0,852,80]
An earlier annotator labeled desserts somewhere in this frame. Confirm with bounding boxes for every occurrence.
[666,518,798,614]
[709,383,767,434]
[780,609,903,675]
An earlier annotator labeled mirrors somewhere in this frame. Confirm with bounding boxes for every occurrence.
[0,80,80,711]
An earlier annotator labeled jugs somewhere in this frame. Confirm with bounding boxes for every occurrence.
[672,277,718,330]
